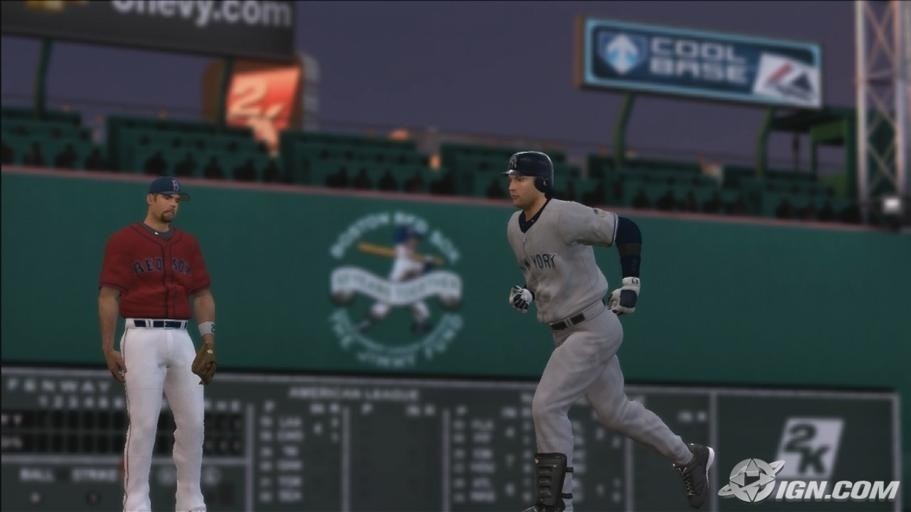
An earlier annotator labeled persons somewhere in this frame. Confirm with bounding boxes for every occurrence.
[499,151,715,512]
[97,176,217,512]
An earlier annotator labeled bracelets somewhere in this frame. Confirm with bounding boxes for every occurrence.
[198,321,217,338]
